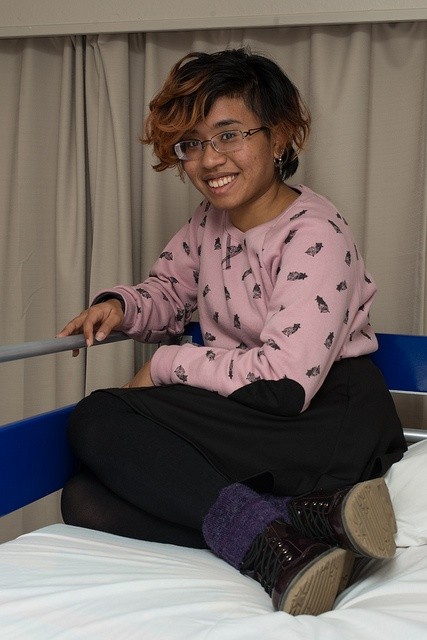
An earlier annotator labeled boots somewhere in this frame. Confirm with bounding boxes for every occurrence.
[260,476,397,573]
[201,482,355,616]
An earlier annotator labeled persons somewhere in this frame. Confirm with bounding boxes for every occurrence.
[56,47,408,615]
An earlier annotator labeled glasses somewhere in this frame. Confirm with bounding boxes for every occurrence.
[172,123,271,162]
